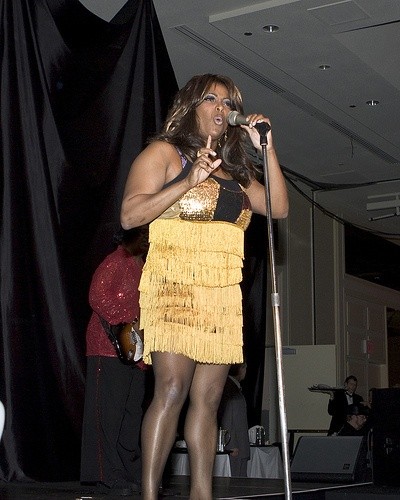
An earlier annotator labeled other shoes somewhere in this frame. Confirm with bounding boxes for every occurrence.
[94,480,132,496]
[128,481,174,495]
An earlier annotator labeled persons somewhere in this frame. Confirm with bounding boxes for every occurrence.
[87,225,153,488]
[327,376,375,484]
[121,74,288,500]
[217,356,250,478]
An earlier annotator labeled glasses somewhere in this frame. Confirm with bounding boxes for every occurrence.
[348,381,357,386]
[240,363,247,368]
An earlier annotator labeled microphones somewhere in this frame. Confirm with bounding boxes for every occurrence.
[226,111,270,129]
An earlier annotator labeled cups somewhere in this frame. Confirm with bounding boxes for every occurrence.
[255,427,265,446]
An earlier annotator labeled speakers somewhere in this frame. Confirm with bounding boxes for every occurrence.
[293,435,366,481]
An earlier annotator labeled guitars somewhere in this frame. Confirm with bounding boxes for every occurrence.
[114,323,144,364]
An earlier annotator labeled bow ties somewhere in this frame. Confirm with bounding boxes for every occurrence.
[346,392,354,398]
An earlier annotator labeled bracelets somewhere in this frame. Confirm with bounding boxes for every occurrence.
[129,316,139,325]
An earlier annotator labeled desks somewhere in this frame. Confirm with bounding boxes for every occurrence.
[170,443,284,479]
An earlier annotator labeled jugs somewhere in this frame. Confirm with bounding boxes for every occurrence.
[216,426,231,454]
